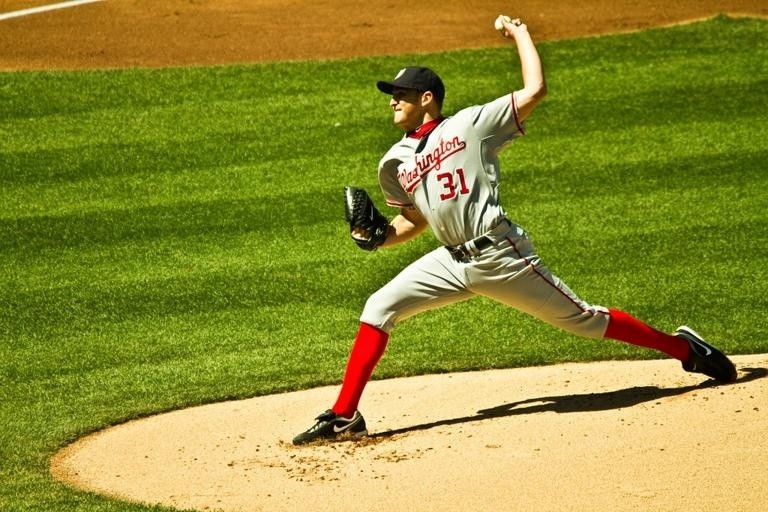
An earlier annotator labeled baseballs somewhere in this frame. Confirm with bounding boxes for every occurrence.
[494,16,513,33]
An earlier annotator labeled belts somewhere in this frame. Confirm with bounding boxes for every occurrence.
[445,220,512,261]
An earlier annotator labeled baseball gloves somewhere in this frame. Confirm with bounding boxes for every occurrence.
[345,186,389,253]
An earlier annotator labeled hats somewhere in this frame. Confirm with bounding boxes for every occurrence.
[377,67,445,99]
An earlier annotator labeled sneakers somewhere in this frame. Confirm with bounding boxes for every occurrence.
[292,411,367,446]
[671,325,739,384]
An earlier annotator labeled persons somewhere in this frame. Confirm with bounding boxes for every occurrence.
[292,14,738,447]
